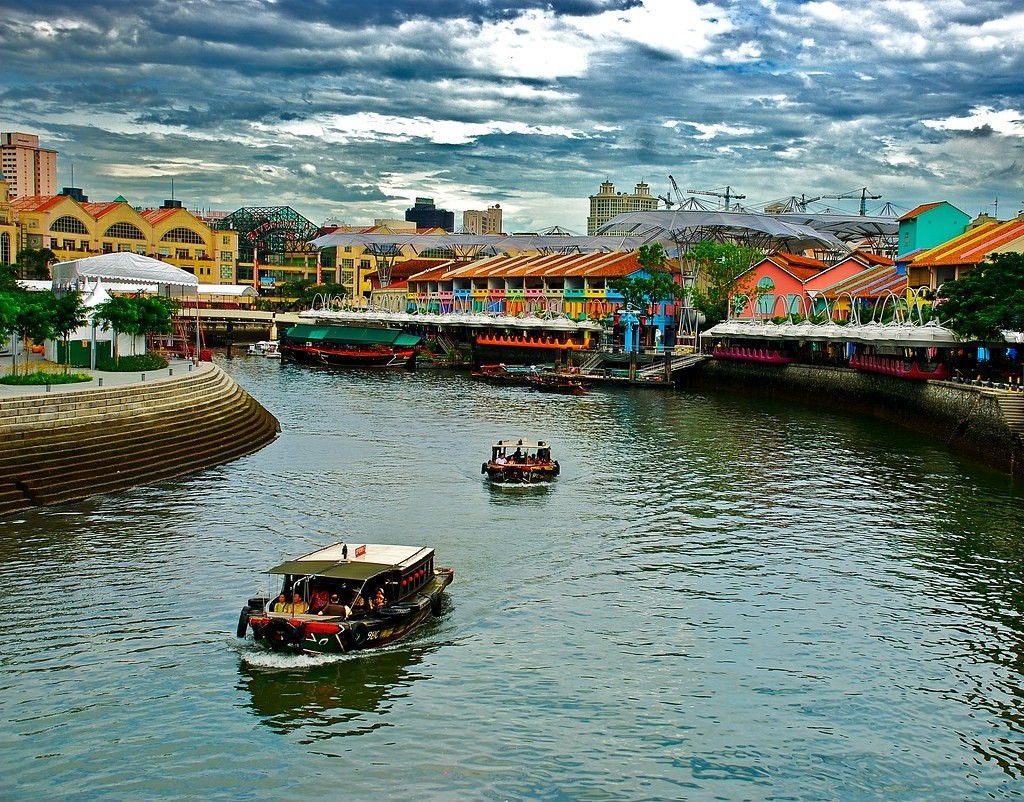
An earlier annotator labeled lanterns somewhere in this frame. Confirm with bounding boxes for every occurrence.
[401,569,425,586]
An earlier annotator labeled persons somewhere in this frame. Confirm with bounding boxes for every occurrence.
[272,580,388,615]
[495,447,551,466]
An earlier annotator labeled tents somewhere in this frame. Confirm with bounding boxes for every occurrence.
[50,251,201,373]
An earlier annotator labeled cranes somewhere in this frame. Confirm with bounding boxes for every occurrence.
[656,175,883,217]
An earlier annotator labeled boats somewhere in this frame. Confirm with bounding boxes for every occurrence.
[469,358,595,397]
[245,321,423,369]
[238,541,454,656]
[482,437,561,485]
